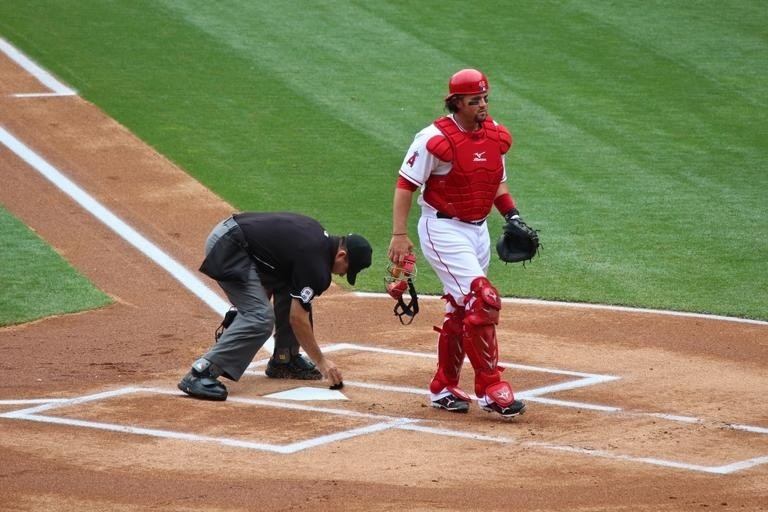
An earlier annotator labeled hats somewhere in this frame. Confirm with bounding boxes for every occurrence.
[347,234,372,286]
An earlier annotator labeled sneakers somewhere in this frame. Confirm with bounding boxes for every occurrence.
[480,400,525,419]
[177,370,227,400]
[430,395,469,411]
[265,357,322,381]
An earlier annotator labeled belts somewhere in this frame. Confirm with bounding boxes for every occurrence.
[436,212,487,226]
[225,218,245,244]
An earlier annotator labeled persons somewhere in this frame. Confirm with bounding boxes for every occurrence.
[176,211,373,401]
[383,69,545,423]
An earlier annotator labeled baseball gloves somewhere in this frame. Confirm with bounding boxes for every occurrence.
[497,208,543,269]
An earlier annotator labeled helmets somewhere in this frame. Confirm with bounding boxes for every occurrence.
[444,69,489,101]
[383,251,417,302]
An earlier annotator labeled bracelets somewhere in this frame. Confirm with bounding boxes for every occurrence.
[391,232,408,236]
